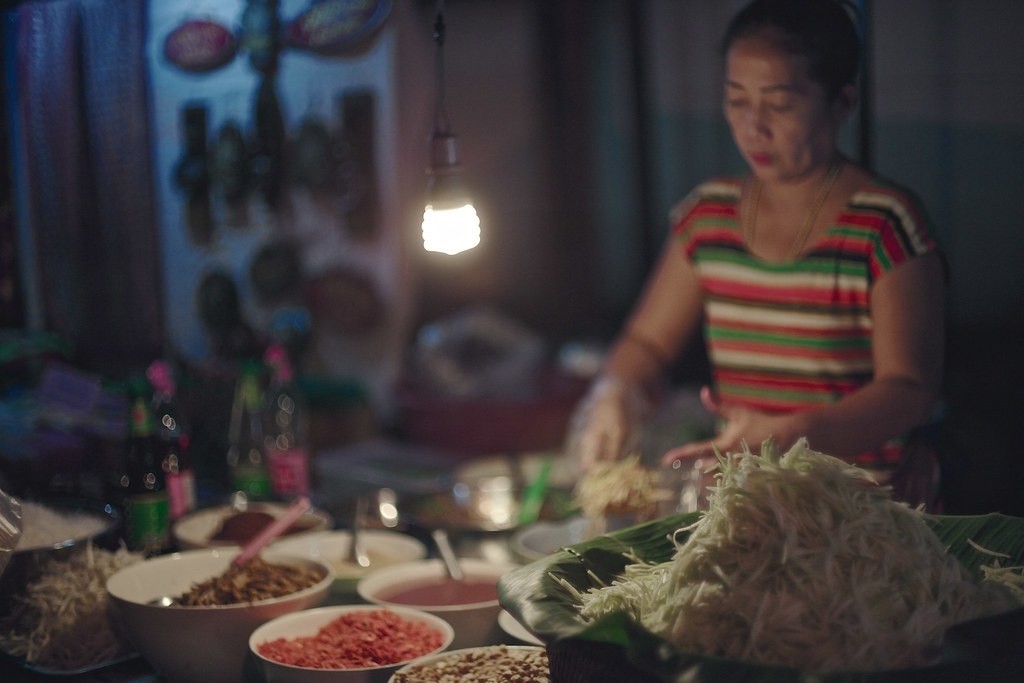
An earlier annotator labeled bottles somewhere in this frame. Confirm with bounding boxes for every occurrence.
[120,376,172,555]
[224,360,270,502]
[146,364,197,516]
[259,346,311,502]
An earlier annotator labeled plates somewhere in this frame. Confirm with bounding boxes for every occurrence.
[20,651,141,675]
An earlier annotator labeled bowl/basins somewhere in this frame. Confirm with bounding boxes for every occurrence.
[106,548,336,683]
[508,517,636,562]
[172,502,333,550]
[388,646,548,683]
[357,557,522,650]
[248,604,455,683]
[0,495,123,604]
[271,528,427,604]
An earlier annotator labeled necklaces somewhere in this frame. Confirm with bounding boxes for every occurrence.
[742,161,844,263]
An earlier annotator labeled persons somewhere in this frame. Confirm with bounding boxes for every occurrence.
[570,0,939,516]
